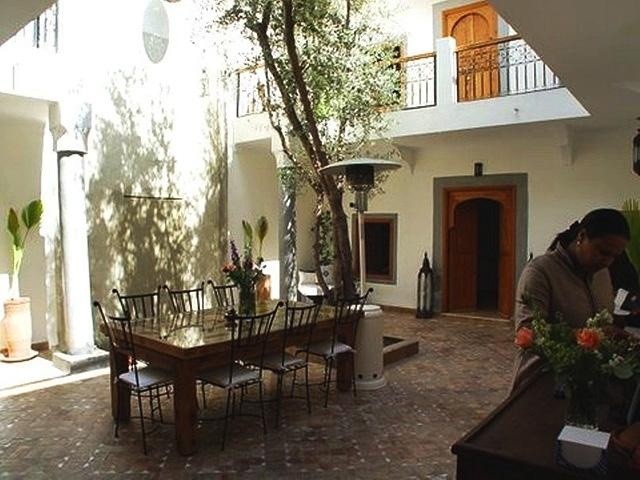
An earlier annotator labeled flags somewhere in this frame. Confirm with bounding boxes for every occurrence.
[238,284,256,314]
[565,385,602,431]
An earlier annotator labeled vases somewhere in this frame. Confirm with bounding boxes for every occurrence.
[512,289,640,390]
[221,216,269,285]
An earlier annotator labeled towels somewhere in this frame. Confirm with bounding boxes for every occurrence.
[347,303,388,391]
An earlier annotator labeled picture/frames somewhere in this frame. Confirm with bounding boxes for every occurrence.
[451,365,640,480]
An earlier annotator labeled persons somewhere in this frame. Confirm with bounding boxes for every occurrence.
[506,206,632,398]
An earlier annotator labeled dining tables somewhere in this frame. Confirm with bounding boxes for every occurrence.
[0,198,43,362]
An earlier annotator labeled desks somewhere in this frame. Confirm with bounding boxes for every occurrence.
[316,157,403,300]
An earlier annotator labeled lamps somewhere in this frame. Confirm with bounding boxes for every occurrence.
[292,287,373,408]
[112,285,201,414]
[195,300,284,452]
[243,298,323,428]
[93,300,174,456]
[207,280,240,307]
[163,282,207,410]
